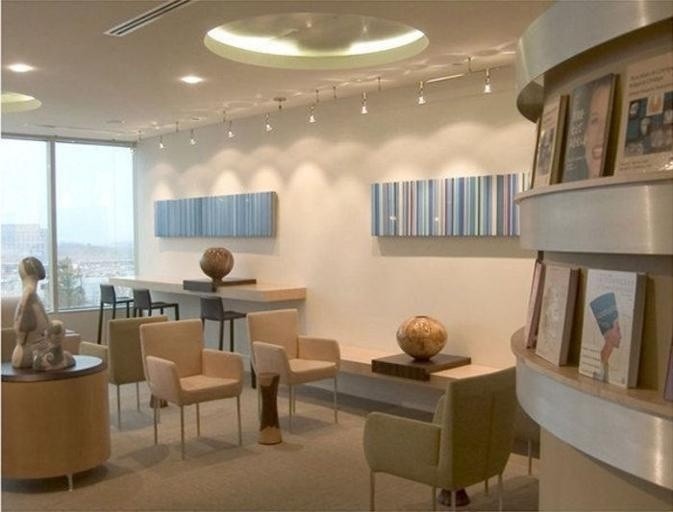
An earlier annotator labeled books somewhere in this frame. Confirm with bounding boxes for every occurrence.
[525,260,673,402]
[528,52,672,189]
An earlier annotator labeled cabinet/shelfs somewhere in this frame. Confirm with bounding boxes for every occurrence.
[505,0,673,487]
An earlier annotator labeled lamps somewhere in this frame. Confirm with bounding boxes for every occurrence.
[155,67,496,151]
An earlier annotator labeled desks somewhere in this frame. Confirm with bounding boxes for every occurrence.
[334,344,504,394]
[109,276,306,303]
[1,354,111,493]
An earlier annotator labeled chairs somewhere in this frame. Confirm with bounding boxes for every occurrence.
[79,316,169,427]
[97,284,142,346]
[199,295,258,389]
[132,288,180,321]
[140,317,245,460]
[1,296,81,364]
[245,308,343,433]
[361,368,519,511]
